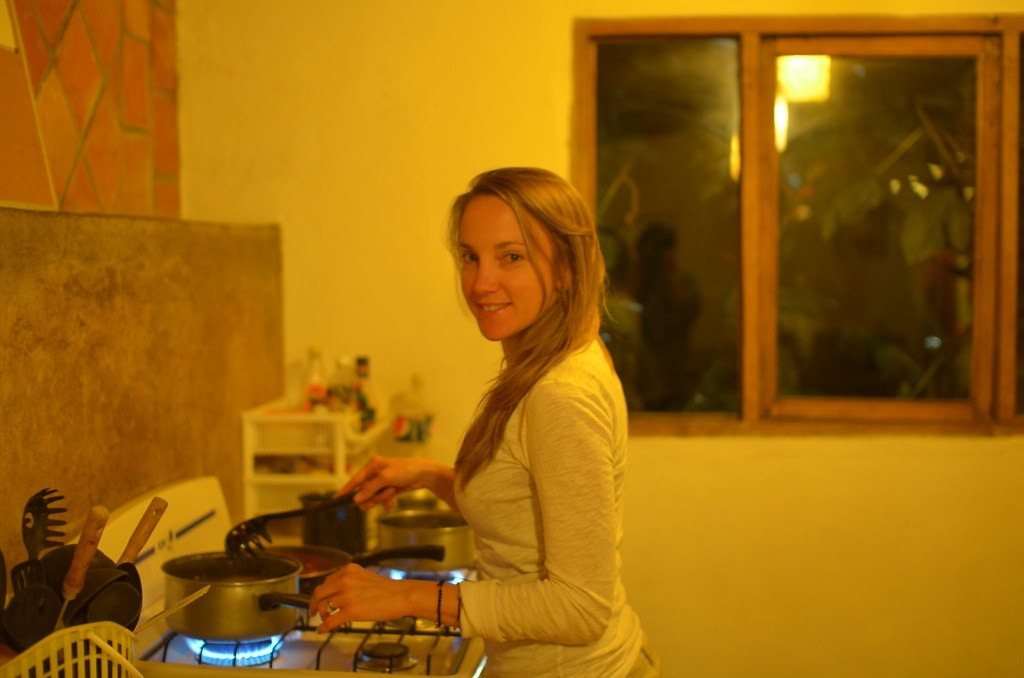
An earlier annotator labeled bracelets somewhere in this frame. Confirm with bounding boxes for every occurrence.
[454,581,464,633]
[437,579,445,629]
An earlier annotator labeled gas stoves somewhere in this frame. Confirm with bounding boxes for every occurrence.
[65,474,484,678]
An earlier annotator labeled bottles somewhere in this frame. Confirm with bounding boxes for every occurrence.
[303,348,382,433]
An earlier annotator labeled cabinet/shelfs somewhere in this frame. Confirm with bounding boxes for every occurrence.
[241,394,391,523]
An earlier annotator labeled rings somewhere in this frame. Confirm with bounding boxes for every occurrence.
[325,596,339,615]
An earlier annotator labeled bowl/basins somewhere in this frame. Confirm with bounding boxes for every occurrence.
[1,544,143,655]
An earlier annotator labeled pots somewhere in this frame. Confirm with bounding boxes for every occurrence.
[162,550,312,640]
[253,545,445,598]
[378,507,477,572]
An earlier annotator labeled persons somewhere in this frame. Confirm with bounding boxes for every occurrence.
[308,166,664,678]
